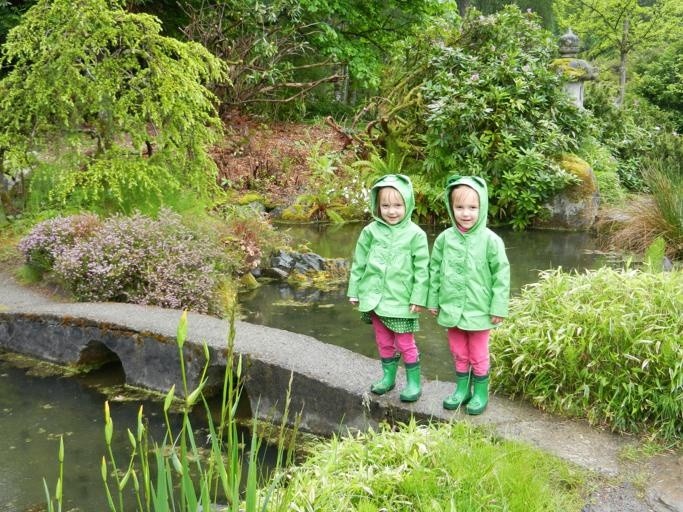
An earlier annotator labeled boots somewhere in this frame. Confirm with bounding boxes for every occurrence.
[370,352,401,395]
[400,360,422,402]
[442,371,473,410]
[465,373,491,415]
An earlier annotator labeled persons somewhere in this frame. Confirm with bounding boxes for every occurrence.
[345,175,430,403]
[425,175,512,413]
